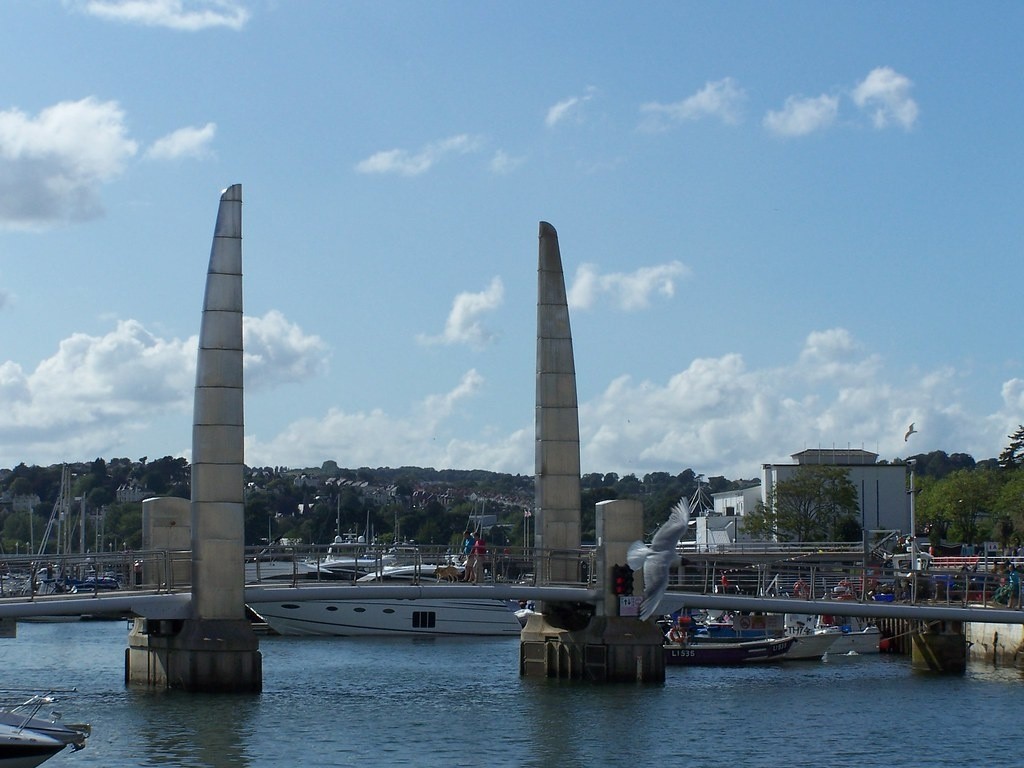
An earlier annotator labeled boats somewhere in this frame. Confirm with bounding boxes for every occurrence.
[0,687,91,768]
[0,461,142,597]
[245,493,499,583]
[245,599,522,636]
[657,608,879,666]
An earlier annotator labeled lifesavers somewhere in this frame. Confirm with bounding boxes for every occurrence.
[837,579,856,601]
[793,579,810,601]
[669,627,686,644]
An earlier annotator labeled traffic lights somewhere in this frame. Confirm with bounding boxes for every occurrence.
[612,563,635,595]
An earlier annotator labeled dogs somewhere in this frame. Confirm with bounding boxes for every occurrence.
[433,567,462,584]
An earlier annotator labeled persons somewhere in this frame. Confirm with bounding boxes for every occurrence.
[990,559,1024,610]
[460,530,476,583]
[468,531,486,585]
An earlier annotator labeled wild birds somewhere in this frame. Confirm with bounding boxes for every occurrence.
[626,496,693,621]
[905,423,917,441]
